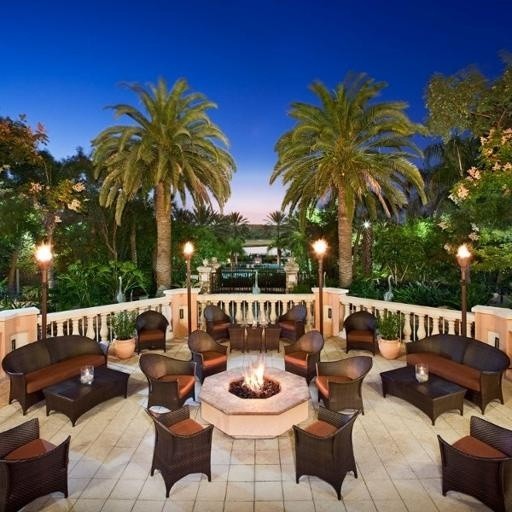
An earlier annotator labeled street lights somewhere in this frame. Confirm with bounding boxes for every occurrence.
[310,238,329,339]
[34,240,54,340]
[455,244,472,338]
[182,241,195,340]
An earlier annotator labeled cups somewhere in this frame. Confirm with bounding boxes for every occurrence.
[415,363,428,382]
[81,365,94,384]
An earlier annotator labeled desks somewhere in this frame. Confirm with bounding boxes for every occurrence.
[226,323,282,354]
[45,368,131,426]
[379,367,466,425]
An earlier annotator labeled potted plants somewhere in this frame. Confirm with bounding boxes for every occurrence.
[110,309,138,358]
[375,313,405,359]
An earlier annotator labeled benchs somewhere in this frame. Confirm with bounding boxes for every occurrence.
[407,335,509,415]
[2,335,109,415]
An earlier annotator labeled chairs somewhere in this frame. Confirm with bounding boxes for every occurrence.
[318,357,372,415]
[292,406,361,499]
[273,304,307,341]
[343,311,378,355]
[436,415,511,510]
[284,330,324,387]
[188,331,228,384]
[205,306,232,340]
[134,311,169,353]
[144,405,214,497]
[138,353,197,411]
[2,419,70,509]
